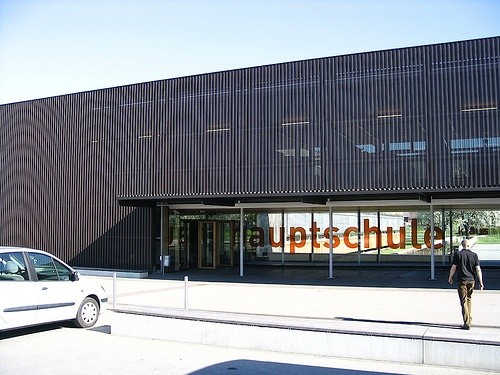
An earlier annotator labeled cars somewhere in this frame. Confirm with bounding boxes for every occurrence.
[0,246,108,333]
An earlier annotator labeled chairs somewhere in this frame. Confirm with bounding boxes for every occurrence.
[0,260,25,281]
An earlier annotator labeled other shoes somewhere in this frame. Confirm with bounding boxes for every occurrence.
[463,325,469,330]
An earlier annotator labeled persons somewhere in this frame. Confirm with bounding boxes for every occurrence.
[448,240,484,331]
